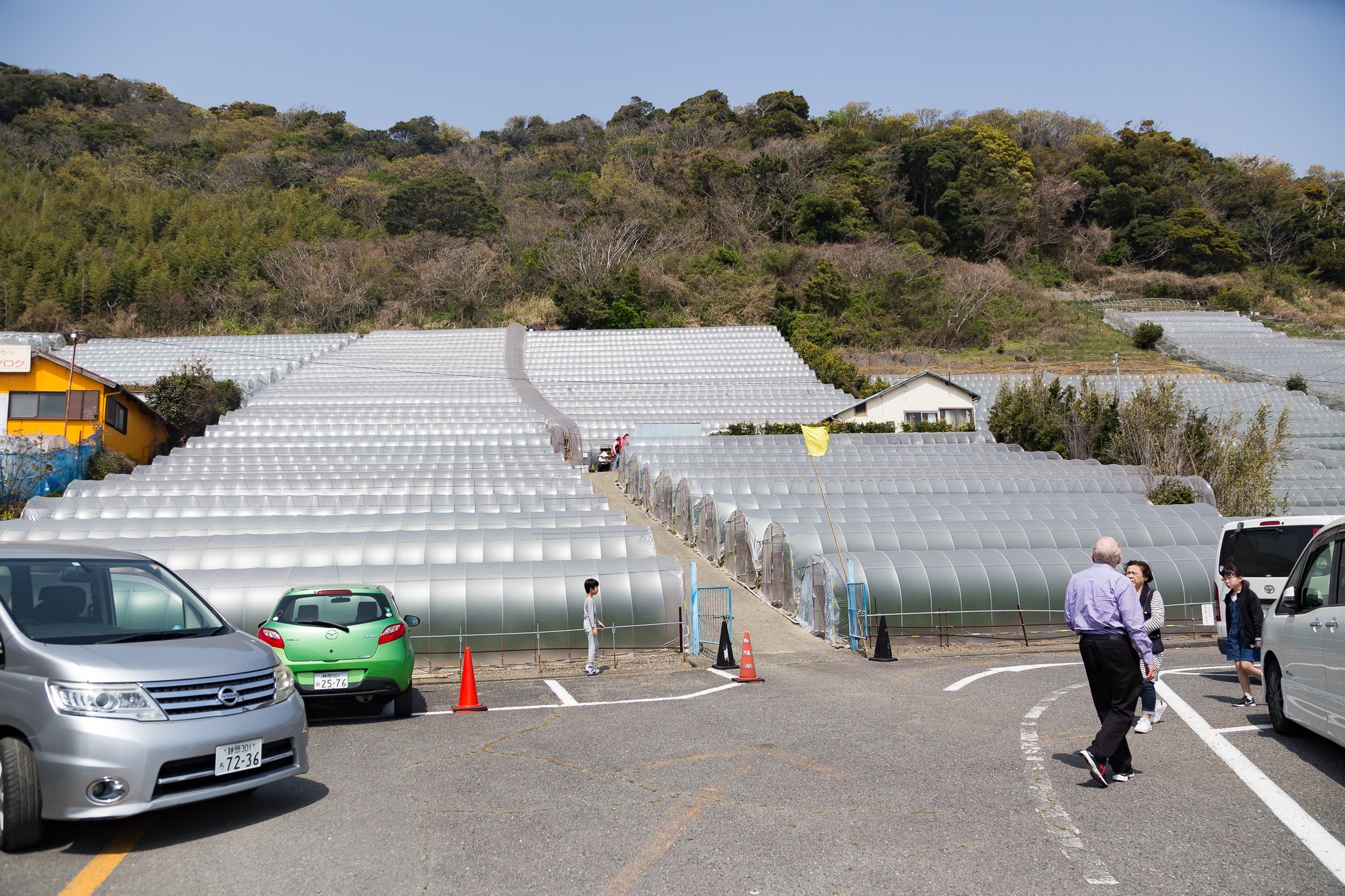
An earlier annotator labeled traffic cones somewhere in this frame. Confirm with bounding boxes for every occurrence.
[449,647,488,711]
[728,631,766,684]
[711,621,742,670]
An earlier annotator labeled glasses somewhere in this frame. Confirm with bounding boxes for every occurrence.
[1221,576,1238,581]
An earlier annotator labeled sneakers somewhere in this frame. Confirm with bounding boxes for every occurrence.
[1231,695,1256,707]
[1134,717,1152,733]
[1150,700,1167,723]
[1078,746,1108,786]
[585,667,599,676]
[1113,767,1134,782]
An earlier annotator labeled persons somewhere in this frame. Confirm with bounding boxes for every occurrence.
[597,452,611,472]
[1221,565,1263,707]
[1063,536,1156,787]
[612,437,620,471]
[618,434,629,456]
[583,578,605,675]
[1122,560,1167,733]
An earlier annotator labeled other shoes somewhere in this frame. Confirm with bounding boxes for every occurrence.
[614,469,619,471]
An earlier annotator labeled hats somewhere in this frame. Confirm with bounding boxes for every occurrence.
[597,452,609,457]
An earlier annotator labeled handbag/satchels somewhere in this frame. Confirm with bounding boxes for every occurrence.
[611,452,616,458]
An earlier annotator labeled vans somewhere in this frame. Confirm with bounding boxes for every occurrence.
[1259,524,1345,750]
[1212,514,1345,653]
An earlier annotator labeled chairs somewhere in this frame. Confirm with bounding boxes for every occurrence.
[355,602,377,622]
[33,586,86,623]
[295,604,318,623]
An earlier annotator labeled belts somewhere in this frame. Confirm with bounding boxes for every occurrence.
[1081,633,1126,641]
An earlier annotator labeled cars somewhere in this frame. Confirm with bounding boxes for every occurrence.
[0,542,312,859]
[256,584,422,718]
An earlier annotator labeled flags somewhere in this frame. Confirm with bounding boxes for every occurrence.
[800,424,830,456]
